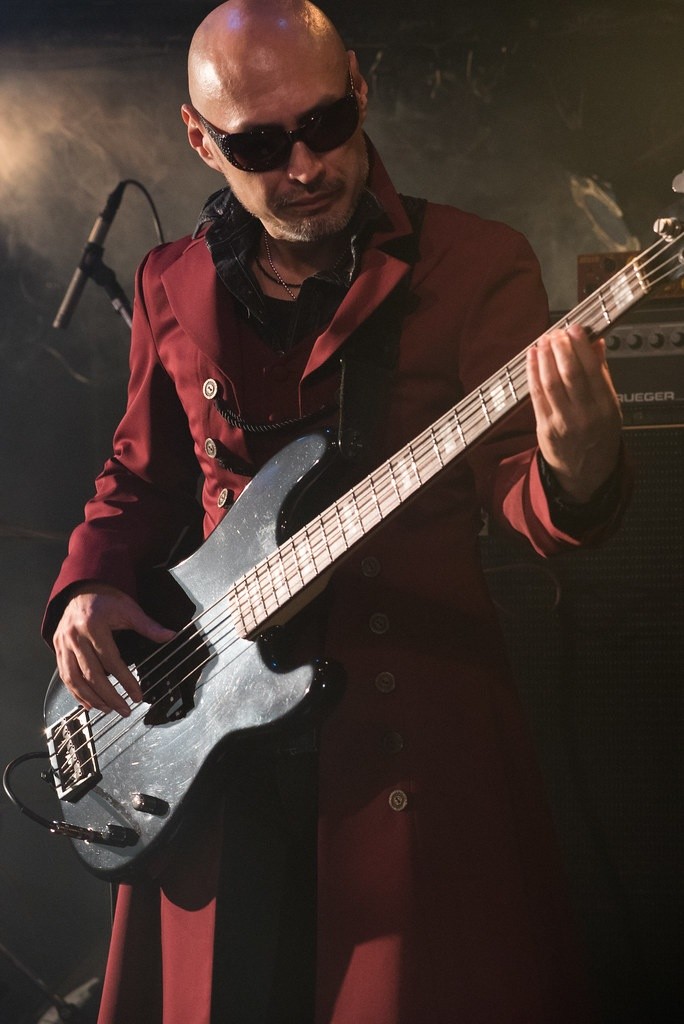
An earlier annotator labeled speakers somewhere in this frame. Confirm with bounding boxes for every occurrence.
[487,427,683,1023]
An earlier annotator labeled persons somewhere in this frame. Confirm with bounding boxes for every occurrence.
[42,0,636,1024]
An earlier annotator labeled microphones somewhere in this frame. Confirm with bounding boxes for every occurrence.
[51,183,129,330]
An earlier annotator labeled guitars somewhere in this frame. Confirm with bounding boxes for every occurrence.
[38,168,683,878]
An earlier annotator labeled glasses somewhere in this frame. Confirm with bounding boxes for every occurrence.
[192,65,359,174]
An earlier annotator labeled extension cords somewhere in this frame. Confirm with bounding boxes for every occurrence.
[39,977,98,1024]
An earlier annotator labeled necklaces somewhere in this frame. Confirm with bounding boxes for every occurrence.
[255,255,350,288]
[263,231,347,299]
